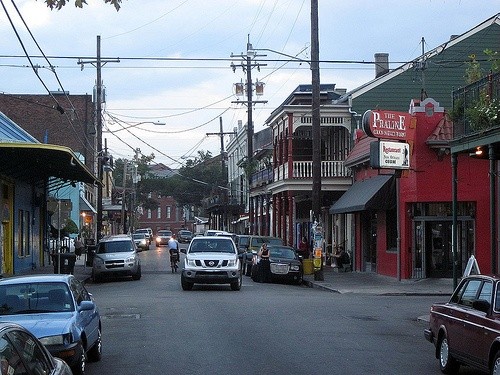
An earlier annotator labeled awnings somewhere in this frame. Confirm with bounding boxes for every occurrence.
[329,175,395,214]
[0,141,104,188]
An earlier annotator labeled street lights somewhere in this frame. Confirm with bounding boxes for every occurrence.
[96,121,166,244]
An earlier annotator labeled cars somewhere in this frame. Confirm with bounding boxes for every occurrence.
[192,233,204,240]
[131,234,150,251]
[0,321,74,375]
[251,243,305,285]
[133,229,152,245]
[0,273,104,375]
[145,228,154,241]
[424,274,500,375]
[102,234,138,252]
[177,231,193,244]
[204,230,255,264]
[155,230,176,247]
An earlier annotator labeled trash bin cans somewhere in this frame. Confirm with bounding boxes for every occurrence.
[87,245,97,266]
[51,253,77,274]
[303,259,314,275]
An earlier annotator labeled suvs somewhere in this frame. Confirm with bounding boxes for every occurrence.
[179,235,245,291]
[239,235,292,276]
[90,237,143,283]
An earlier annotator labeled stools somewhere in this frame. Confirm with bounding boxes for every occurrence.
[343,264,350,272]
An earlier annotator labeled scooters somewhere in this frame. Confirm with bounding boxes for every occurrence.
[169,245,181,274]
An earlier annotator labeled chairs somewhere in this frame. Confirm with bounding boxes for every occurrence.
[196,242,229,250]
[49,289,66,307]
[2,295,25,312]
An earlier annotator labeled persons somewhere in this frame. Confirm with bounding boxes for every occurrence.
[74,237,83,261]
[257,243,270,284]
[168,235,181,267]
[299,236,350,269]
